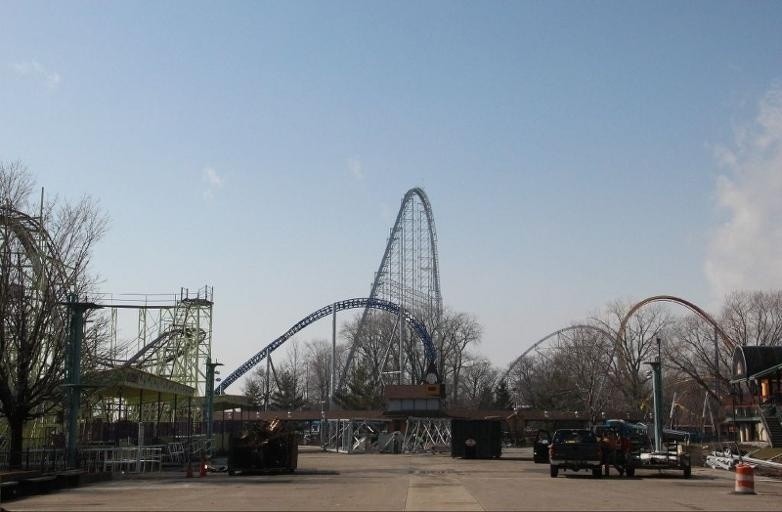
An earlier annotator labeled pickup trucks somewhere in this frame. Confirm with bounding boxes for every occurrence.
[531,429,613,478]
